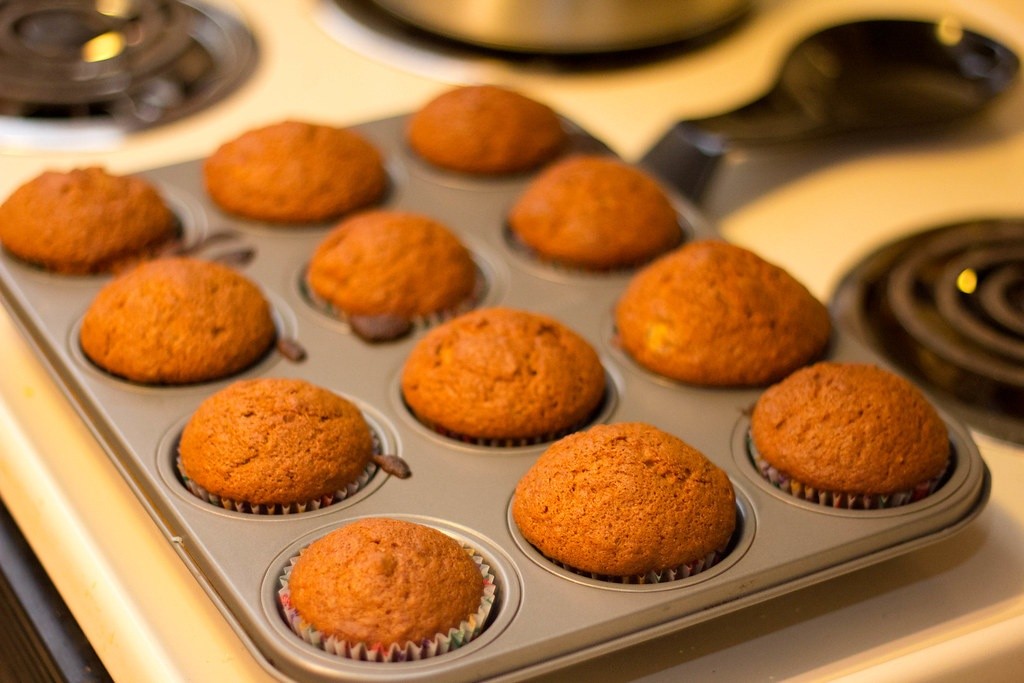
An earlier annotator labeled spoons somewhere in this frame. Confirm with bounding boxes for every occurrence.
[643,17,1021,201]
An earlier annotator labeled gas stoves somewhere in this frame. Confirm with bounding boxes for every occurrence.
[0,0,261,152]
[830,212,1024,449]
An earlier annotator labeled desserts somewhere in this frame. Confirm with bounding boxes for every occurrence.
[0,85,951,655]
